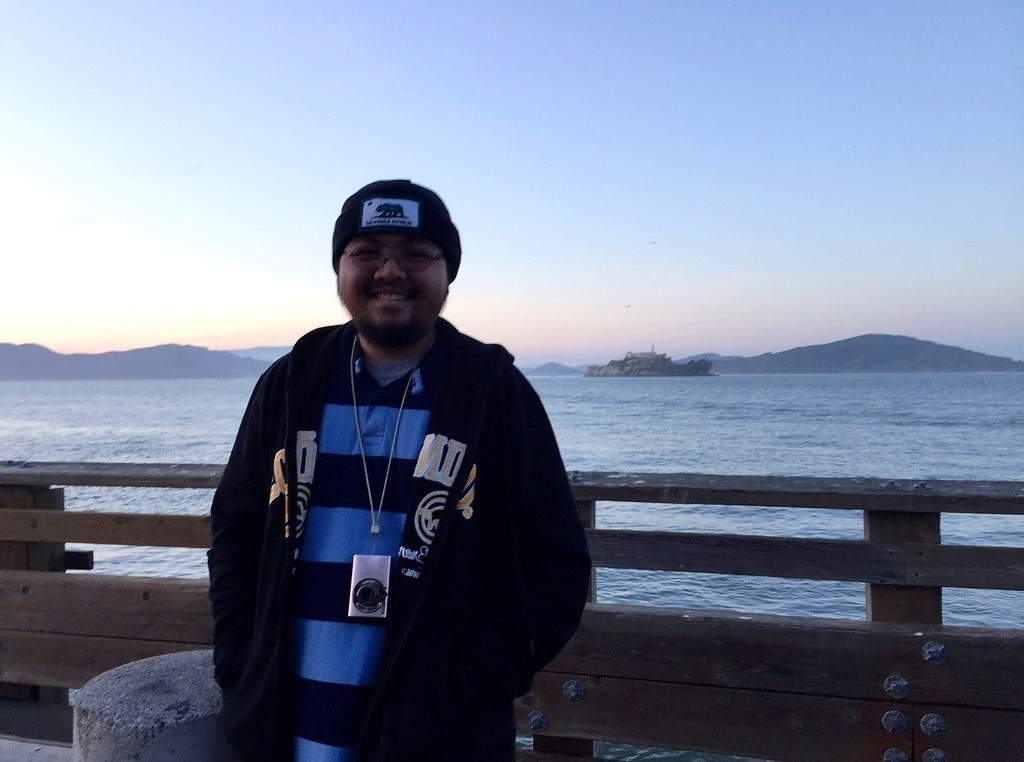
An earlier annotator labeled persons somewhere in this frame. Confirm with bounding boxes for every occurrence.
[206,179,590,762]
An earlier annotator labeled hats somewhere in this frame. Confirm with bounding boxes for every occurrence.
[332,180,461,285]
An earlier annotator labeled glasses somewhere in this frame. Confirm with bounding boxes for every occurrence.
[344,248,442,270]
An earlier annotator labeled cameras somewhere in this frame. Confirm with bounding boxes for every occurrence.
[348,554,391,617]
